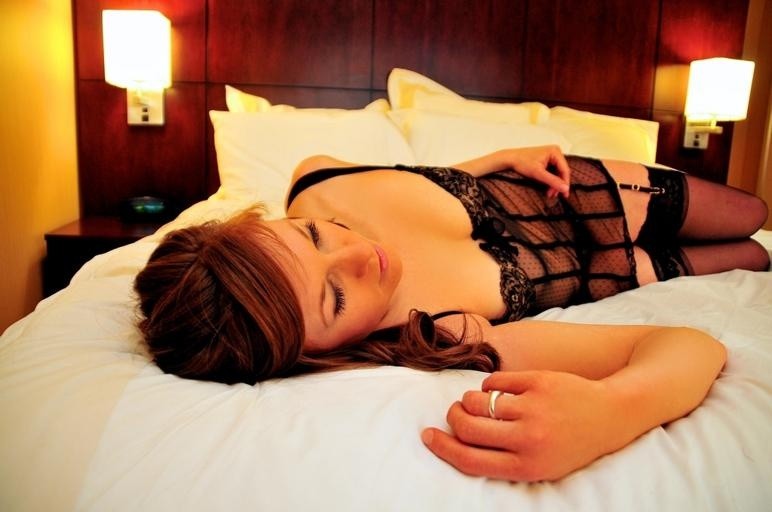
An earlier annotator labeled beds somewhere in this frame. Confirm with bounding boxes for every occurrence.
[2,187,772,512]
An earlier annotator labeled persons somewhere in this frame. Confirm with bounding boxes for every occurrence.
[134,145,770,483]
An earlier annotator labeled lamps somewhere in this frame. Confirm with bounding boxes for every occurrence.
[101,7,173,126]
[683,58,756,149]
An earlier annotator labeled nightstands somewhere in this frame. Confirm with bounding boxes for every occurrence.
[42,216,172,299]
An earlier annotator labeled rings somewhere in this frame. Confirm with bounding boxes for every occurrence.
[488,390,503,419]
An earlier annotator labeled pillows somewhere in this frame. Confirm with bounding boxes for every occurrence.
[208,65,662,212]
